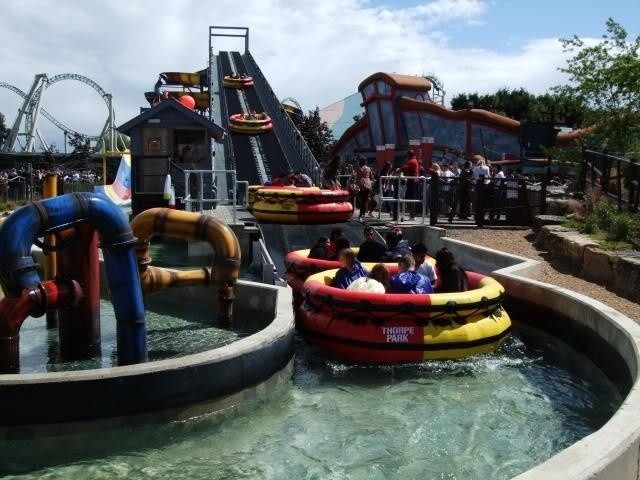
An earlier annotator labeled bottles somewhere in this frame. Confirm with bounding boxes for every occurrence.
[392,227,402,234]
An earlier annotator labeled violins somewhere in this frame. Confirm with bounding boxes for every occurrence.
[145,48,325,197]
[92,149,132,207]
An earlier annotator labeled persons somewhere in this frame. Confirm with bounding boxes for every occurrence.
[385,231,410,260]
[357,227,386,262]
[307,235,331,260]
[252,110,258,120]
[347,264,392,294]
[240,114,247,120]
[230,72,248,79]
[248,114,256,120]
[261,112,266,120]
[163,90,169,99]
[329,238,350,261]
[326,228,345,257]
[411,243,438,289]
[432,247,468,293]
[333,248,370,289]
[180,146,196,164]
[386,254,434,294]
[0,166,101,201]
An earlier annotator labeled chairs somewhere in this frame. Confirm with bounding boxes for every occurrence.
[404,217,416,221]
